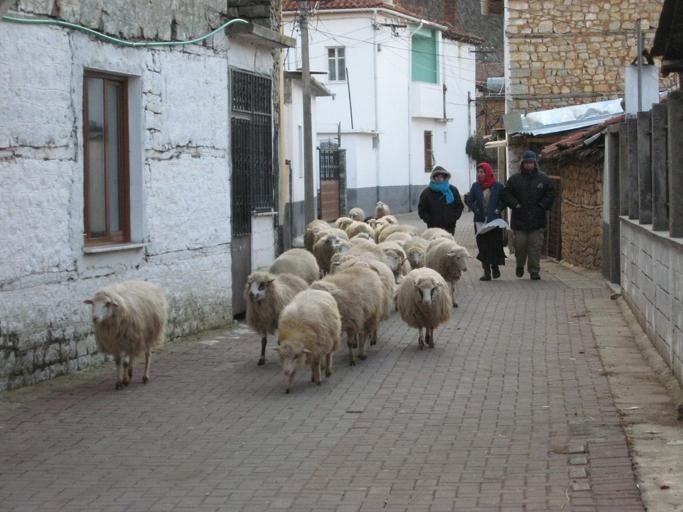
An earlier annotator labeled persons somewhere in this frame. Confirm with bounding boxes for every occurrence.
[463,161,505,281]
[500,149,555,280]
[416,165,464,237]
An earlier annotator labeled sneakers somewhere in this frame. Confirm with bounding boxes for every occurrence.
[479,265,500,281]
[515,265,541,280]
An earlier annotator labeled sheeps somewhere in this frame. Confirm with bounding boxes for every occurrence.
[349,207,366,222]
[375,200,389,218]
[84,280,170,391]
[243,214,472,398]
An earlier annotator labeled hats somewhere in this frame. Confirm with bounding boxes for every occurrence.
[519,150,540,170]
